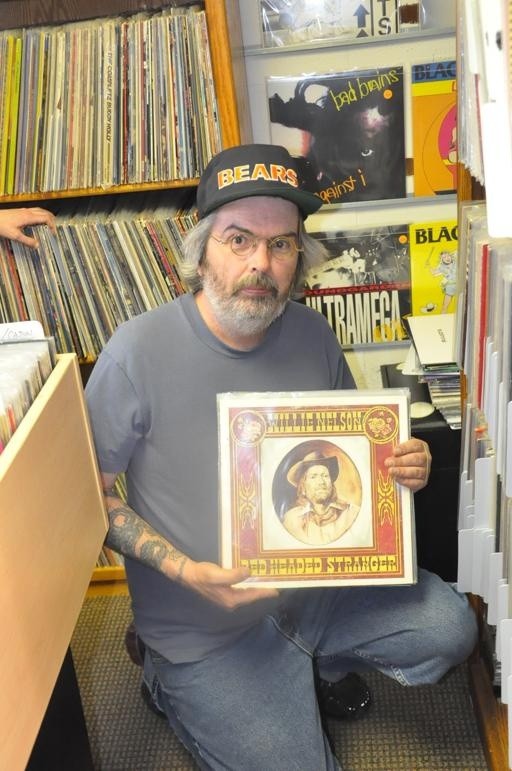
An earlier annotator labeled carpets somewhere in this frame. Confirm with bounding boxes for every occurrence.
[73,596,491,771]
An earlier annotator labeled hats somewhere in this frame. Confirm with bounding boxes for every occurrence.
[286,451,340,485]
[196,145,323,220]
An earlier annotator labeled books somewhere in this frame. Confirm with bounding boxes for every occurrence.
[1,320,59,454]
[405,312,465,431]
[261,1,401,51]
[289,222,411,353]
[1,6,224,192]
[455,200,512,708]
[410,219,459,316]
[266,67,411,205]
[0,196,204,369]
[411,59,458,199]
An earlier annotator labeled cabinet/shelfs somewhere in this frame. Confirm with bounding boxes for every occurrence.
[1,0,253,584]
[452,0,509,771]
[2,351,110,769]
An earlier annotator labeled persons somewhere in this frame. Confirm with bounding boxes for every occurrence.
[1,207,61,252]
[281,451,360,544]
[80,145,480,769]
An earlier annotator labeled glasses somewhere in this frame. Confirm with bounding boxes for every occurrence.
[209,232,304,261]
